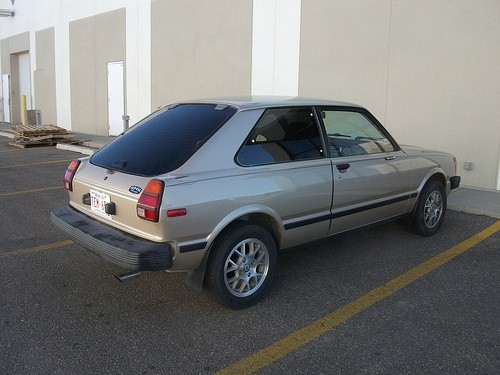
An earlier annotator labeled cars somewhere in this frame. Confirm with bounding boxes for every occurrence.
[48,93,462,310]
